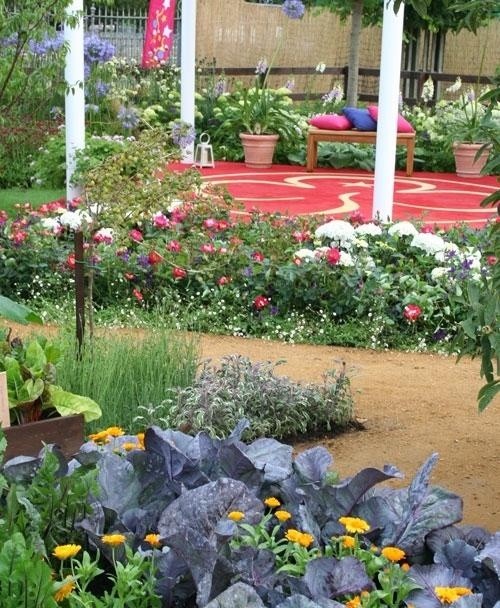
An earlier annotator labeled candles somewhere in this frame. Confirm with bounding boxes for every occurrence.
[201,150,208,164]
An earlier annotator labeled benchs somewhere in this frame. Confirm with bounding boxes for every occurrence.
[307,129,416,176]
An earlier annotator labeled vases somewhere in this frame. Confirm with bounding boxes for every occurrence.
[453,145,492,178]
[239,134,281,169]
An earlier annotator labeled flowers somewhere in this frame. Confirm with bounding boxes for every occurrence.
[215,0,305,134]
[444,90,500,144]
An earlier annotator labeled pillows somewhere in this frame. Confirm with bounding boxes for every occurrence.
[368,106,413,133]
[310,114,351,130]
[341,107,376,132]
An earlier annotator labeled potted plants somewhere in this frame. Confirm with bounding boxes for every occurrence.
[0,337,102,461]
